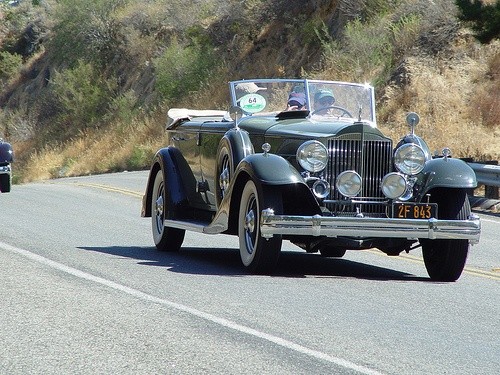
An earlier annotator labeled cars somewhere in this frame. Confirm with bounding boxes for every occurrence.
[0,138,13,192]
[140,78,482,282]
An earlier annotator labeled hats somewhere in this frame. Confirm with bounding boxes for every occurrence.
[314,88,335,104]
[287,92,307,106]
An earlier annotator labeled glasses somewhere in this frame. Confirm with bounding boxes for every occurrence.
[318,98,334,105]
[288,103,303,108]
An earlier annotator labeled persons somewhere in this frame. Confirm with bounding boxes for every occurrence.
[284,86,308,111]
[313,88,335,115]
